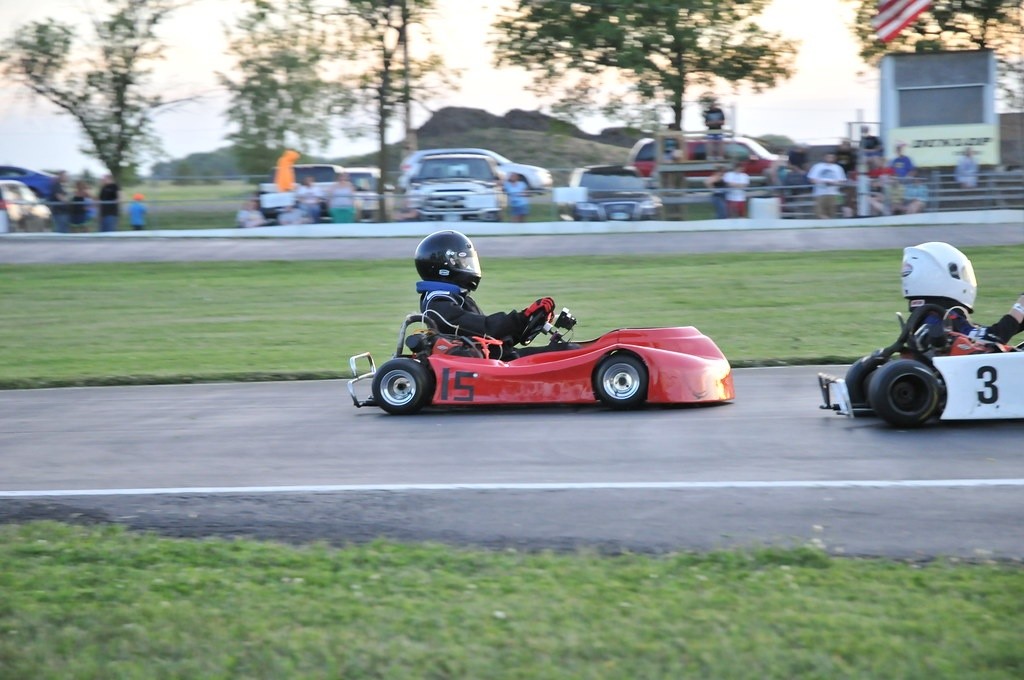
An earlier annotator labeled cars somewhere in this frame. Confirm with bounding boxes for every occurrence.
[559,165,667,221]
[252,164,385,222]
[1,176,51,233]
[396,153,511,222]
[628,132,794,192]
[395,147,555,190]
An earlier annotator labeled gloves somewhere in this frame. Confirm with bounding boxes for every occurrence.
[523,297,554,320]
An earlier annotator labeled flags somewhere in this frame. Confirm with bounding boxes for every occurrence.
[870,0,937,43]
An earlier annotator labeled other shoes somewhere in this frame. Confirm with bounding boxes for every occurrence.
[706,155,724,163]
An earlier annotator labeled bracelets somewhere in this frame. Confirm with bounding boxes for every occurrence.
[1012,302,1024,316]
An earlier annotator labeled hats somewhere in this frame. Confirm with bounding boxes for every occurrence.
[133,193,144,199]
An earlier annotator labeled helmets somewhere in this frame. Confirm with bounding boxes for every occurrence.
[901,241,977,313]
[415,230,482,290]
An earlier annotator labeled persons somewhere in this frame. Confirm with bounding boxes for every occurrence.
[235,173,420,227]
[954,147,981,191]
[416,231,582,363]
[126,193,150,230]
[49,172,123,234]
[661,102,929,219]
[500,173,532,222]
[902,242,1024,356]
